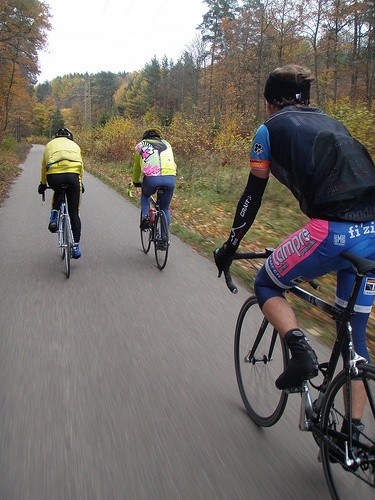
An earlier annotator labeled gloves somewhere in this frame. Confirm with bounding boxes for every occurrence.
[133,182,142,187]
[215,242,239,272]
[38,183,47,194]
[82,185,85,193]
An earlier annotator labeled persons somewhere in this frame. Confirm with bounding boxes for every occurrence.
[38,128,84,258]
[131,127,177,250]
[213,64,375,471]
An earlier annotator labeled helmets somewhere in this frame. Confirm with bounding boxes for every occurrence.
[142,128,164,140]
[55,128,73,141]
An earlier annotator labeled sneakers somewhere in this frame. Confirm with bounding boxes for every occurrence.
[72,244,81,258]
[329,439,359,463]
[48,209,59,232]
[275,350,319,390]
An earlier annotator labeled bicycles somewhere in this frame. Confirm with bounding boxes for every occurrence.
[127,181,171,271]
[214,245,375,500]
[41,184,85,278]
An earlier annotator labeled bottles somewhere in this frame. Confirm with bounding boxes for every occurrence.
[149,208,155,222]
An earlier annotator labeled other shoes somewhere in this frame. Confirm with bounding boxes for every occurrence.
[140,219,151,231]
[156,241,170,250]
